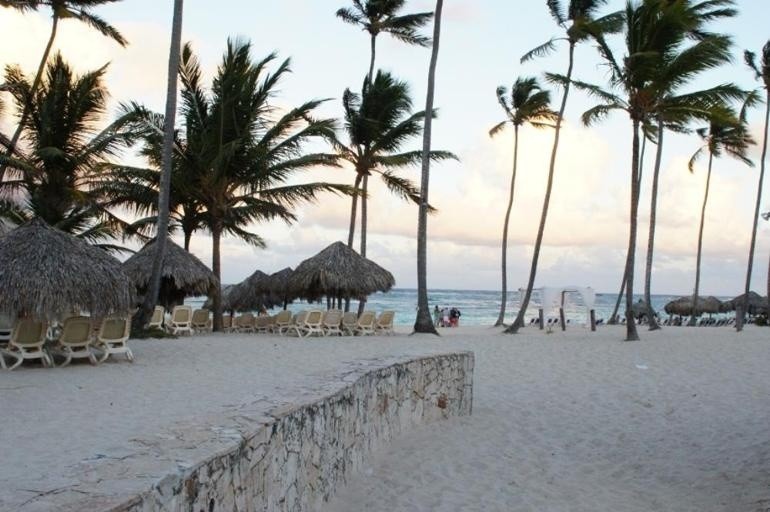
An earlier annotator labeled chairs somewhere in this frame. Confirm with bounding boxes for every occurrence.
[213,305,396,341]
[133,306,213,339]
[0,311,135,372]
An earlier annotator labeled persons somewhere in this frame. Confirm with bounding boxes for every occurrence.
[432,304,461,328]
